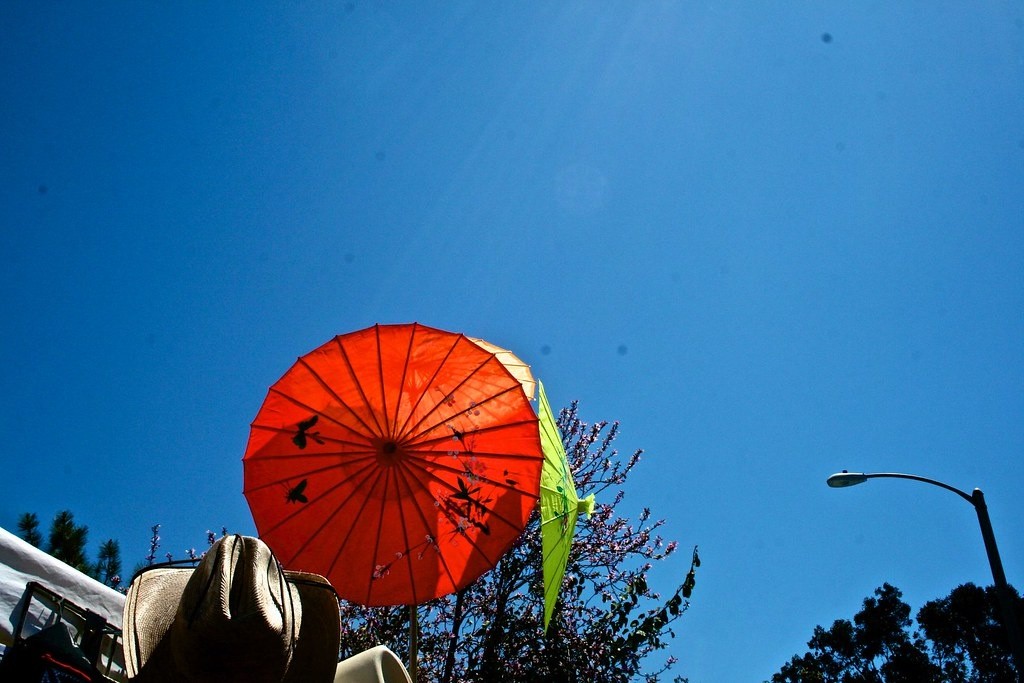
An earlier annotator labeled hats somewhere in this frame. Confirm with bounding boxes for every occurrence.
[331,638,413,683]
[121,533,342,683]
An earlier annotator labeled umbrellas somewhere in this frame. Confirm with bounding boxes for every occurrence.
[466,336,536,404]
[536,378,595,634]
[242,320,545,611]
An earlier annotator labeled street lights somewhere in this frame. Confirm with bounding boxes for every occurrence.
[826,469,1008,587]
